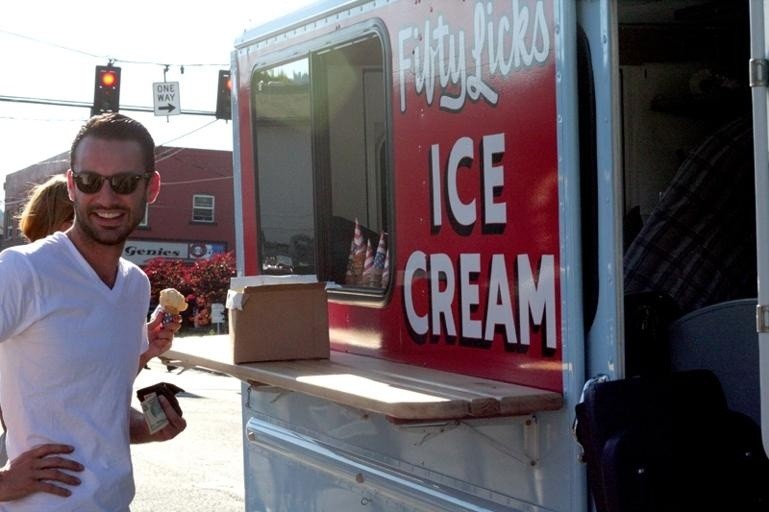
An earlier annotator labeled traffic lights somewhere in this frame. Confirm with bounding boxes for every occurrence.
[92,65,121,116]
[217,70,231,120]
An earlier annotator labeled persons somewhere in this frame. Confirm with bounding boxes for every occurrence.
[19,172,185,379]
[1,112,190,512]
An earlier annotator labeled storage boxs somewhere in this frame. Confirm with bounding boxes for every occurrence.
[227,271,331,366]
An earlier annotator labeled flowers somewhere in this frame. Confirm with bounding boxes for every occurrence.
[141,249,238,330]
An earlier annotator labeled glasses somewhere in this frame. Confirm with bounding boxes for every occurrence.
[71,168,151,195]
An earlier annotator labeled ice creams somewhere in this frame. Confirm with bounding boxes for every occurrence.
[159,288,188,326]
